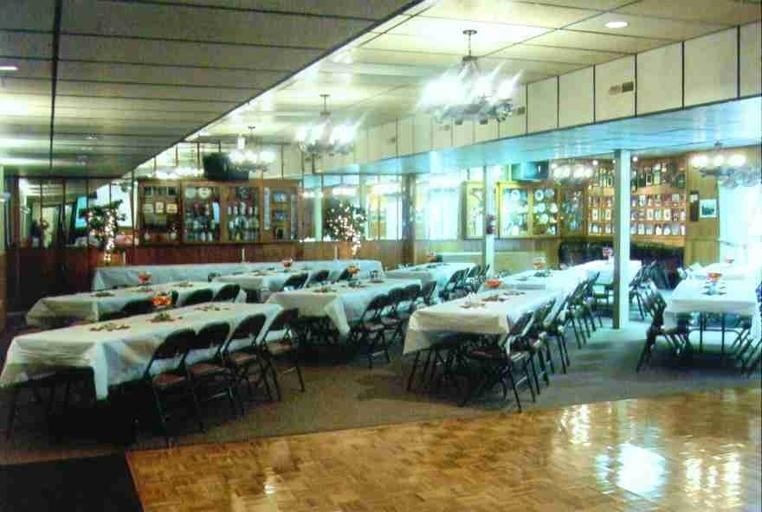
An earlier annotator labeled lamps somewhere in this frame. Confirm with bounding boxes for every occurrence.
[415,28,524,127]
[548,158,599,189]
[225,124,276,174]
[293,92,364,159]
[685,150,748,180]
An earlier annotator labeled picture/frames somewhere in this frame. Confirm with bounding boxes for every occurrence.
[697,197,719,220]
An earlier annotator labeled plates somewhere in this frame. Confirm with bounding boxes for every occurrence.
[535,190,544,202]
[537,203,545,213]
[511,189,520,201]
[545,188,554,198]
[538,214,548,224]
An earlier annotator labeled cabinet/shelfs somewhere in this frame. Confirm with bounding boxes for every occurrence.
[586,153,721,241]
[459,179,485,242]
[134,177,302,247]
[5,237,424,314]
[485,180,587,273]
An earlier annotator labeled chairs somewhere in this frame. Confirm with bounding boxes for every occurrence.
[438,263,492,303]
[403,283,421,315]
[580,270,604,335]
[311,267,330,288]
[512,293,558,395]
[344,293,391,370]
[96,310,128,322]
[180,286,216,308]
[187,321,240,435]
[222,312,274,419]
[456,309,537,416]
[629,259,697,375]
[376,287,405,349]
[336,265,358,283]
[212,282,242,303]
[119,297,154,317]
[420,280,438,307]
[544,291,572,377]
[122,326,209,450]
[556,279,588,350]
[253,306,307,404]
[279,272,309,347]
